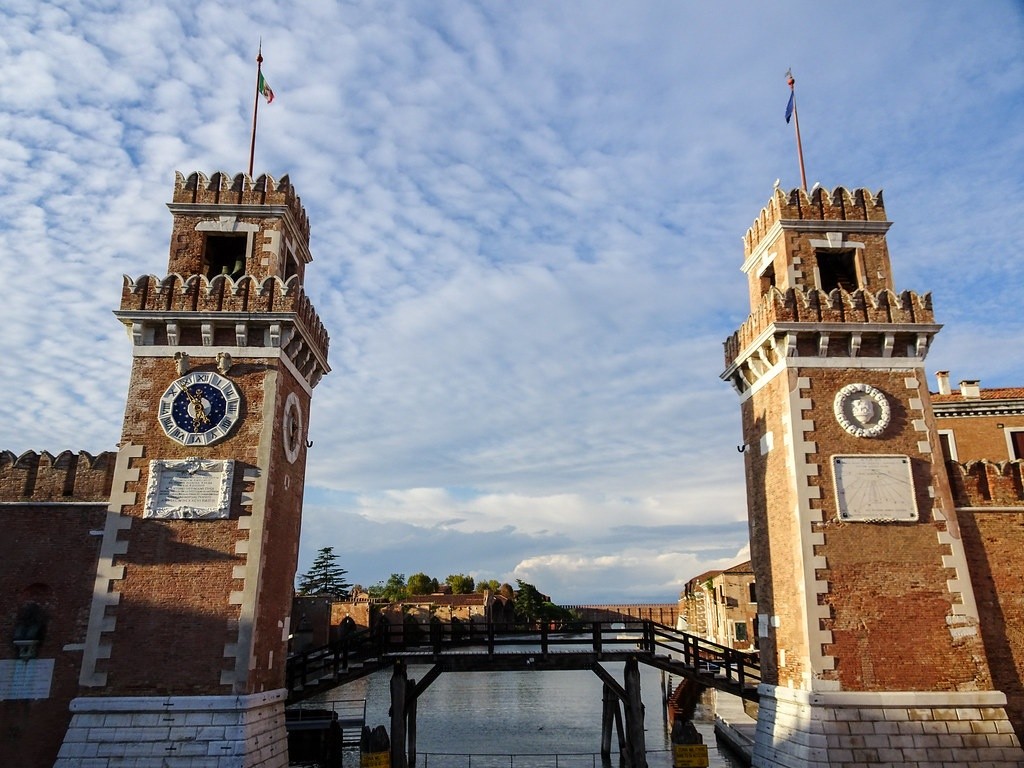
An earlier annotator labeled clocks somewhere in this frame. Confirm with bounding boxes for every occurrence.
[157,372,241,447]
[282,391,303,464]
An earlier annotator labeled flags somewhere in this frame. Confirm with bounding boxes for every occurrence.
[258,71,275,104]
[785,92,793,124]
